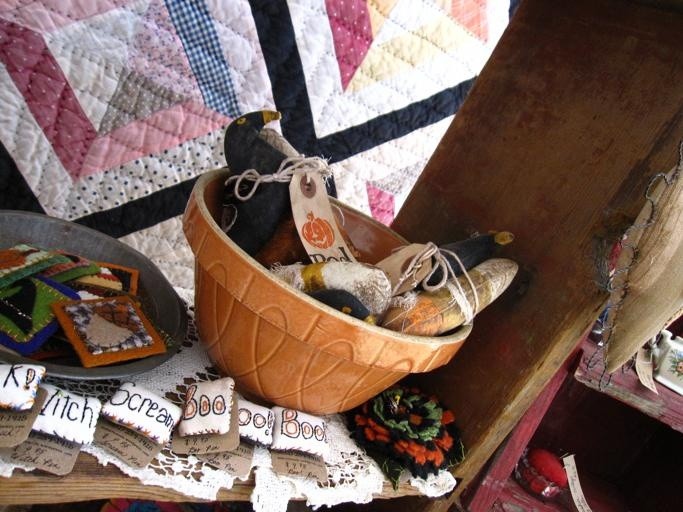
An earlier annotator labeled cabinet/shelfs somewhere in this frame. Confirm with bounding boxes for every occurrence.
[459,326,683,512]
[0,1,683,512]
